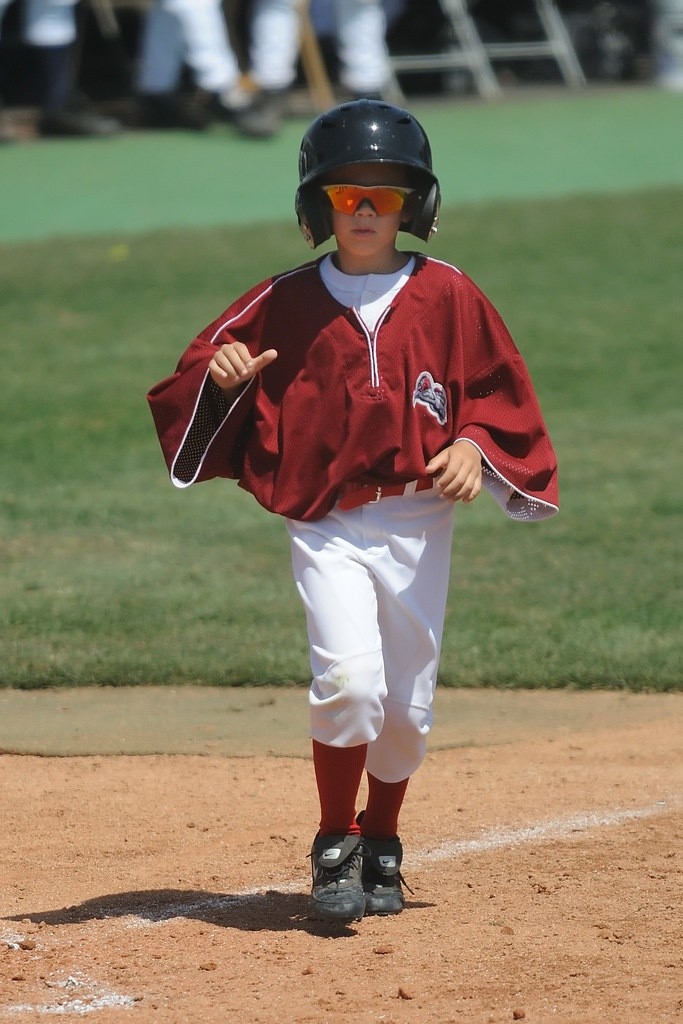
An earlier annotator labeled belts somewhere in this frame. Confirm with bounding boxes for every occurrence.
[336,478,433,511]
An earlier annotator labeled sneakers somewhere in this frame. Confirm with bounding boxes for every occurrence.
[305,828,366,919]
[355,809,415,916]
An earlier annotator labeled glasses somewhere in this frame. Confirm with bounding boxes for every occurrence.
[319,183,419,216]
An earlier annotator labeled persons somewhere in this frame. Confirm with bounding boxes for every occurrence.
[0,0,394,142]
[146,98,559,921]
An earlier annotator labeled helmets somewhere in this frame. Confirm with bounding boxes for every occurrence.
[295,97,442,248]
